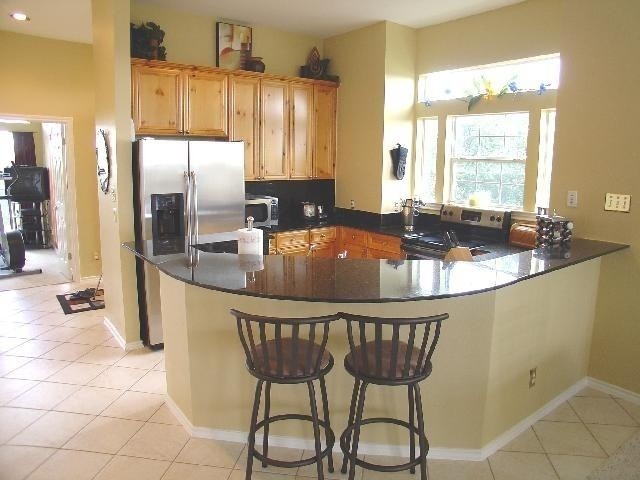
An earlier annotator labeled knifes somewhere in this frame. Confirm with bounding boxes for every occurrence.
[440,229,462,254]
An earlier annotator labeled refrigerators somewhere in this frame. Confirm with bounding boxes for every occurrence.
[132,135,245,347]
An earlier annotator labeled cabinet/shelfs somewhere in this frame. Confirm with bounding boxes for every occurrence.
[276,227,337,257]
[228,70,339,182]
[131,57,228,139]
[337,224,403,260]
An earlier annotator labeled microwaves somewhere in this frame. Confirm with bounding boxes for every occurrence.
[244,194,279,228]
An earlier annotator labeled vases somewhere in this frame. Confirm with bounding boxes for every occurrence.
[247,56,265,73]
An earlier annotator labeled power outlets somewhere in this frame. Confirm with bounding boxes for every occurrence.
[604,193,631,212]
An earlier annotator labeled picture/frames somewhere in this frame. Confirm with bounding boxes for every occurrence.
[216,22,252,70]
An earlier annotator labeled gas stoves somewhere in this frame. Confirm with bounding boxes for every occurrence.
[400,203,511,256]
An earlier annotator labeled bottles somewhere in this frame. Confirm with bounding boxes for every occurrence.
[402,199,419,232]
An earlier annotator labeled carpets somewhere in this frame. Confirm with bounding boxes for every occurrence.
[57,289,105,314]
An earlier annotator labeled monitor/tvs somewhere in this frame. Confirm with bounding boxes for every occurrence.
[3,167,49,199]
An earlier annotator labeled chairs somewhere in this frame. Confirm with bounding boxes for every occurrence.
[228,305,450,480]
